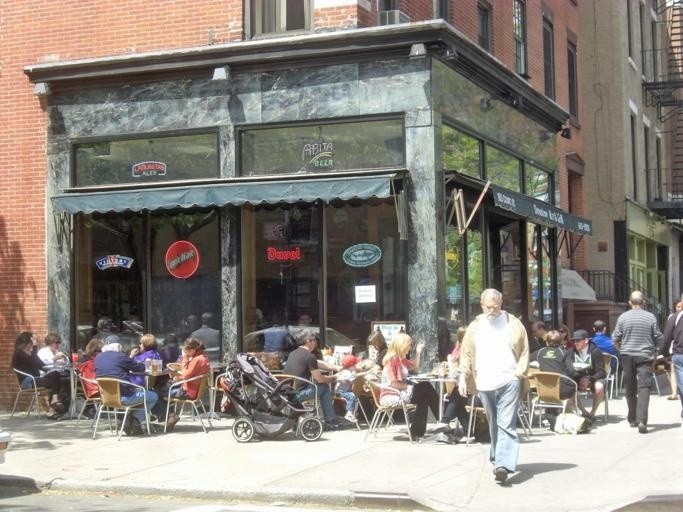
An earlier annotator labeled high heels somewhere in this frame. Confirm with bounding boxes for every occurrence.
[325,422,334,428]
[331,422,342,427]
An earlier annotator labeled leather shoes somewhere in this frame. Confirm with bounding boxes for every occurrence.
[490,458,495,465]
[495,467,507,484]
[450,419,455,422]
[408,434,424,440]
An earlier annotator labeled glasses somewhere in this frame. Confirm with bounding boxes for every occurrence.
[53,340,63,344]
[184,346,193,350]
[558,329,567,333]
[324,345,329,350]
[138,342,144,345]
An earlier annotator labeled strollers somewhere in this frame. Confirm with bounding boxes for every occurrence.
[219,352,323,443]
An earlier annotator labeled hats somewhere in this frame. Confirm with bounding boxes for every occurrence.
[104,334,122,344]
[569,329,590,341]
[342,354,360,368]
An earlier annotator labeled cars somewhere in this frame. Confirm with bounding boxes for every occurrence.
[203,325,365,360]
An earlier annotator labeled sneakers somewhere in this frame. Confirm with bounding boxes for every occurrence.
[456,436,475,443]
[354,412,358,418]
[638,422,648,433]
[630,421,637,427]
[428,421,450,434]
[344,411,358,423]
[129,415,144,435]
[121,415,133,435]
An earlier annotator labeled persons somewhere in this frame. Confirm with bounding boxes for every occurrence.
[531,320,549,350]
[79,339,103,397]
[666,299,682,400]
[334,355,362,424]
[293,311,320,351]
[379,333,444,441]
[255,315,294,353]
[564,329,606,423]
[658,293,682,415]
[184,312,223,364]
[94,335,158,435]
[91,317,131,354]
[157,335,182,386]
[11,331,64,421]
[129,333,162,387]
[530,330,576,429]
[457,288,530,484]
[555,325,575,354]
[151,338,210,432]
[430,326,476,443]
[356,330,389,423]
[612,290,664,432]
[38,332,70,382]
[280,327,346,430]
[35,333,72,412]
[588,320,619,373]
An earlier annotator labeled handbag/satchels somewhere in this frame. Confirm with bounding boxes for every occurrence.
[174,384,189,400]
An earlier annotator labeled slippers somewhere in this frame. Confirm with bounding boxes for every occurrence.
[48,401,65,411]
[45,413,62,420]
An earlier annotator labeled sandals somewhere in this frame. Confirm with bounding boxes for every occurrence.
[166,425,176,433]
[667,395,678,400]
[581,411,589,418]
[588,414,597,420]
[165,414,180,429]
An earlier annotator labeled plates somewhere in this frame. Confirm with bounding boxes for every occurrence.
[428,372,450,379]
[409,375,435,380]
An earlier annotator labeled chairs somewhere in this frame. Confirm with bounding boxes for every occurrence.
[93,378,150,437]
[366,372,419,443]
[585,353,619,400]
[523,372,580,435]
[210,373,242,420]
[273,375,320,437]
[579,352,611,422]
[332,374,370,431]
[166,363,183,379]
[466,373,534,445]
[75,368,101,429]
[163,373,212,434]
[637,348,672,397]
[11,368,52,419]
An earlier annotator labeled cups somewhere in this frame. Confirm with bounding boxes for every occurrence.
[433,363,439,371]
[145,359,153,373]
[73,354,80,368]
[448,363,457,379]
[44,359,49,366]
[158,361,163,372]
[334,355,341,368]
[151,360,158,374]
[321,350,330,363]
[438,364,446,381]
